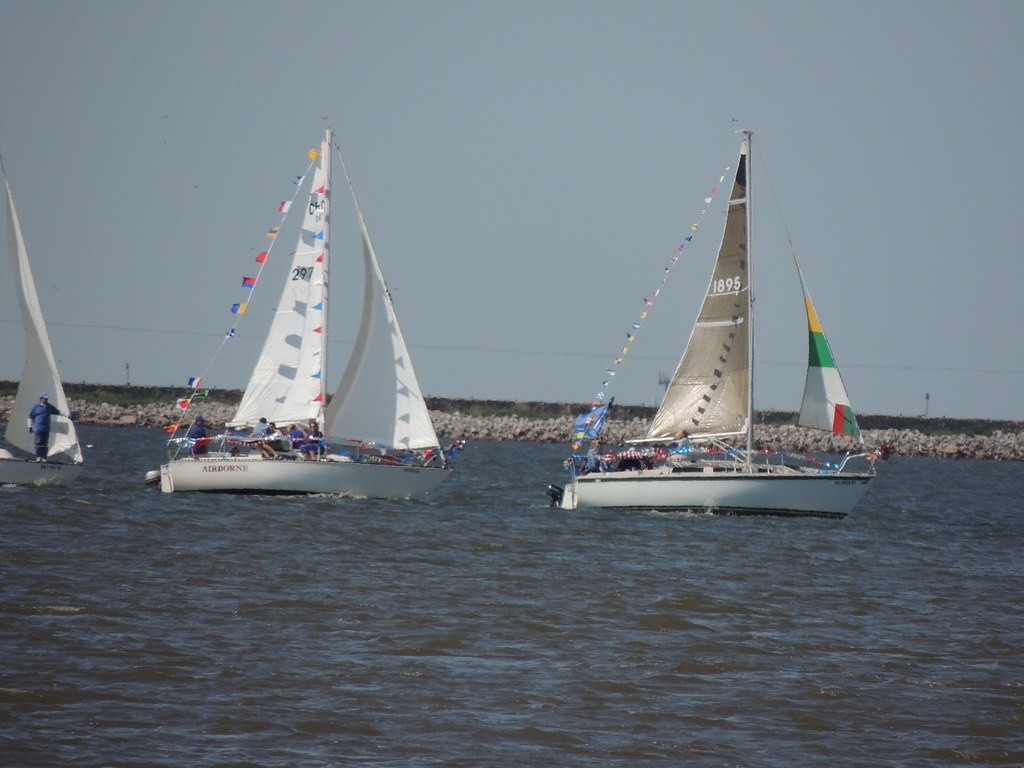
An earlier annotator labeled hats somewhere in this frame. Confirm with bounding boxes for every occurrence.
[676,430,688,439]
[39,392,50,399]
[195,416,206,422]
[595,440,603,446]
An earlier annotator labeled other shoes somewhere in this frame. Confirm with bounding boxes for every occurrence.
[42,458,47,463]
[267,456,273,460]
[36,457,41,462]
[275,455,283,461]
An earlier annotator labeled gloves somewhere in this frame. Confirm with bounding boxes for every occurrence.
[29,427,34,434]
[68,415,74,420]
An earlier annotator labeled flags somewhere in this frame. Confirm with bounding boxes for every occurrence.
[242,276,256,288]
[164,423,178,432]
[176,398,190,410]
[256,252,267,263]
[227,327,235,337]
[573,405,607,438]
[194,390,209,399]
[188,378,202,388]
[232,303,248,314]
[267,226,279,238]
[294,176,305,186]
[309,148,318,161]
[279,201,292,213]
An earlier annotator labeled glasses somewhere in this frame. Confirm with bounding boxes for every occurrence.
[310,426,315,428]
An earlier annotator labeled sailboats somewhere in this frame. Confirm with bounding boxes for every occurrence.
[0,163,96,490]
[546,123,900,521]
[144,129,468,504]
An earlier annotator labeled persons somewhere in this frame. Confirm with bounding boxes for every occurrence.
[679,430,690,448]
[253,417,280,459]
[28,393,72,462]
[643,462,652,470]
[287,425,304,450]
[301,423,325,461]
[189,416,208,455]
